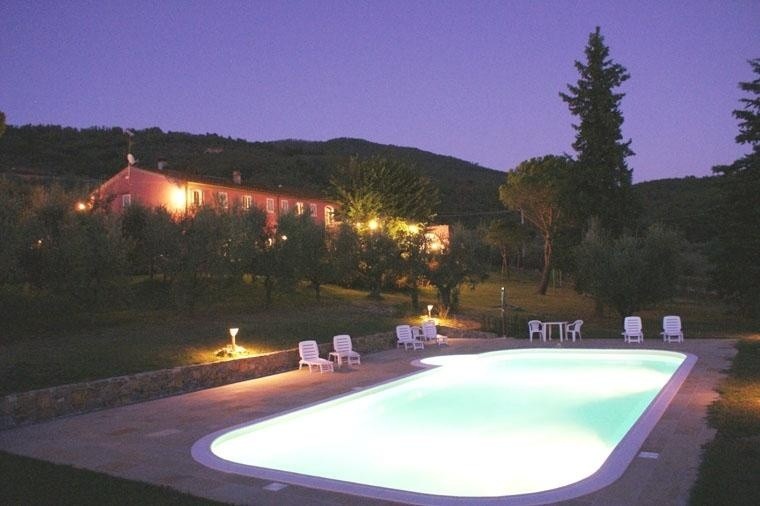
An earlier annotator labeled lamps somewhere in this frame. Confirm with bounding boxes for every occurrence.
[427,304,434,319]
[229,326,240,351]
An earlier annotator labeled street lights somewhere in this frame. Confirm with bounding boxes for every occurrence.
[500,285,507,337]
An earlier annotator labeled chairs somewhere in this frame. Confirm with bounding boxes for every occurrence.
[422,321,447,346]
[621,316,644,344]
[661,315,685,344]
[566,319,583,342]
[396,324,424,350]
[334,334,361,365]
[528,319,546,342]
[298,340,334,374]
[410,326,424,341]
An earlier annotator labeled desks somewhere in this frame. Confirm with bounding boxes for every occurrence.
[542,321,569,342]
[328,351,351,368]
